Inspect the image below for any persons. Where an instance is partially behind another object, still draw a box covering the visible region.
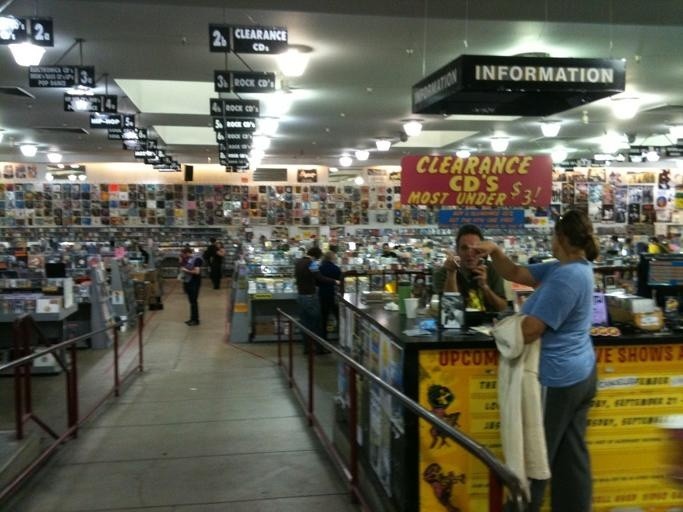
[317,251,345,338]
[215,241,225,279]
[176,246,203,326]
[293,246,340,356]
[381,242,397,257]
[203,237,224,289]
[465,207,602,512]
[432,224,508,314]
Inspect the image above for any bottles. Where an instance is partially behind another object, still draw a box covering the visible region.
[397,280,411,314]
[430,294,439,311]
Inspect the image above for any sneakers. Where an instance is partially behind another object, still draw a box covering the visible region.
[185,319,200,326]
[316,348,331,355]
[305,349,313,355]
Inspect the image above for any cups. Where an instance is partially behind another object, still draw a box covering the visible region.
[402,297,420,319]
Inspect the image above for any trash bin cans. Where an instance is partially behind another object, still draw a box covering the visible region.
[63,303,91,348]
